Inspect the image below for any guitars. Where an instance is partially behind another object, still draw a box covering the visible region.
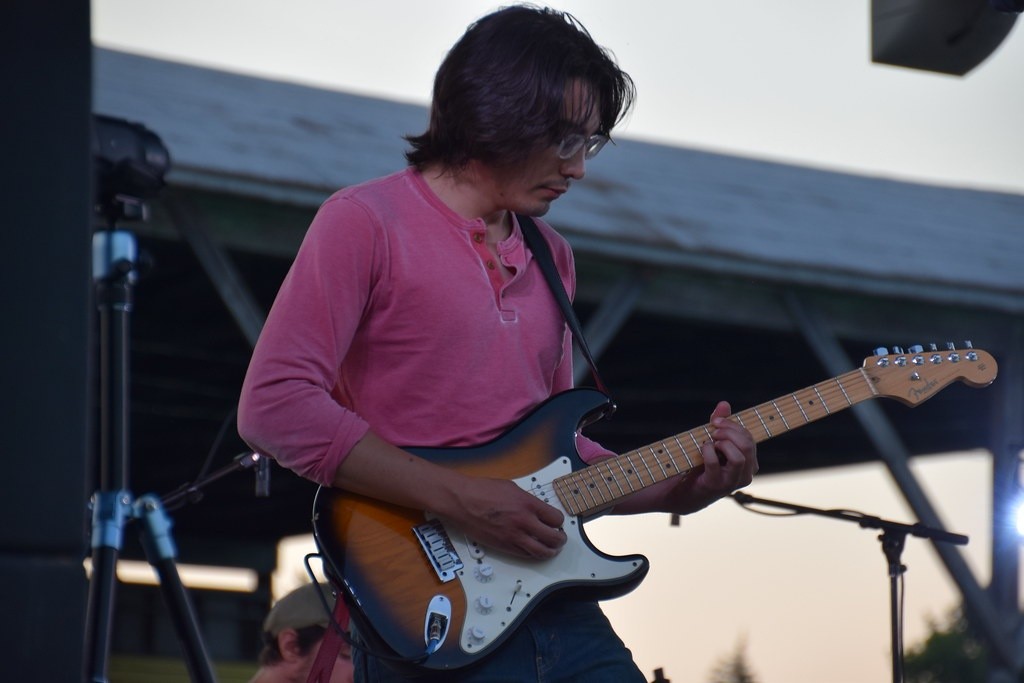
[312,340,997,681]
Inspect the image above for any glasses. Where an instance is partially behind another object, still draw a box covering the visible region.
[556,133,608,159]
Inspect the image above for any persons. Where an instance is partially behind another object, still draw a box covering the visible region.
[219,4,763,683]
[239,578,375,682]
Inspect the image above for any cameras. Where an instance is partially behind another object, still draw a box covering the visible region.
[89,113,168,209]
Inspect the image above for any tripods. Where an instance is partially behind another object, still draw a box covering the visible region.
[81,230,220,683]
